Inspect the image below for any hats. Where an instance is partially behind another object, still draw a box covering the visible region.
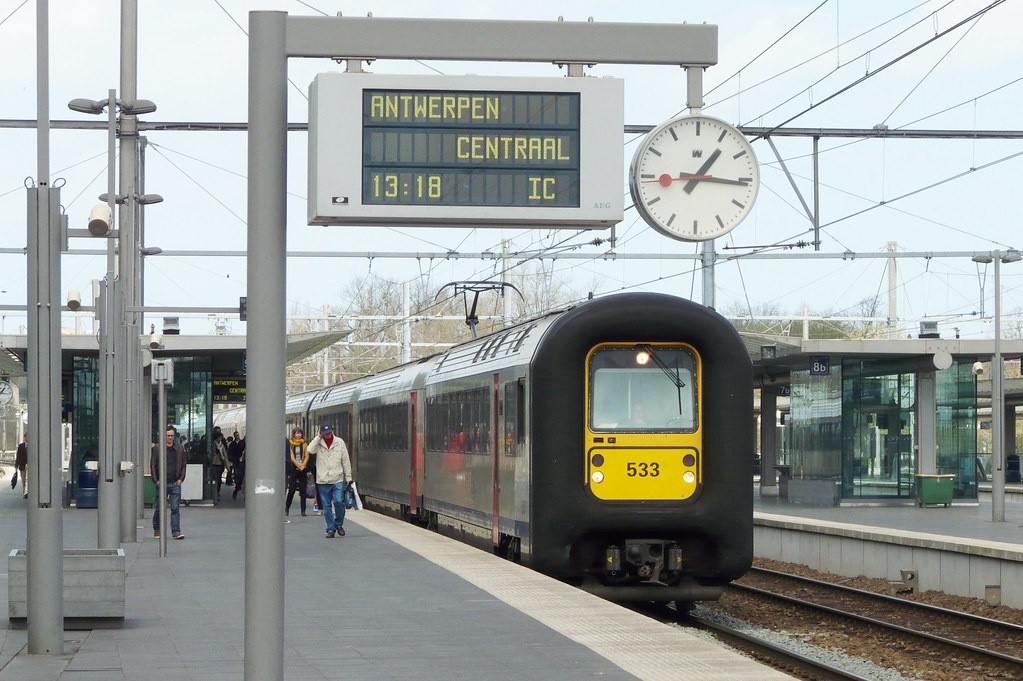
[320,423,332,434]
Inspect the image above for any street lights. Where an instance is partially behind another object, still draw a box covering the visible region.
[67,88,165,552]
[972,248,1022,522]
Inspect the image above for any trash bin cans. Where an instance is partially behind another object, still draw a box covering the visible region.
[143,476,156,508]
[914,473,956,508]
[753,458,761,474]
[1007,455,1021,482]
[76,470,98,509]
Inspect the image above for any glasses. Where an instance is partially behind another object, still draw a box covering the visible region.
[167,435,173,437]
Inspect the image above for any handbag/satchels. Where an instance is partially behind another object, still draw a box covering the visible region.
[226,471,233,486]
[350,481,363,511]
[239,452,245,475]
[345,482,354,509]
[11,472,17,489]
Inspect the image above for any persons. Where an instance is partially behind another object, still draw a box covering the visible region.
[15,432,28,498]
[308,453,324,516]
[151,427,186,539]
[214,426,245,501]
[306,425,353,538]
[175,429,209,483]
[285,427,311,516]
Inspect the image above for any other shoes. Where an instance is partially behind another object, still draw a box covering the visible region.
[233,492,236,499]
[23,495,27,498]
[285,511,289,516]
[313,504,318,511]
[301,511,306,516]
[316,510,324,515]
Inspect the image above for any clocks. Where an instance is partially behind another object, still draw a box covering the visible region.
[630,113,761,242]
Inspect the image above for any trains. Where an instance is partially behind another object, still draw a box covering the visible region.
[176,281,755,618]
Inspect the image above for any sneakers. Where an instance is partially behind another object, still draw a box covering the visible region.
[173,531,184,539]
[154,530,160,538]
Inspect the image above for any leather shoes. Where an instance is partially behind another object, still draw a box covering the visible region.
[336,527,345,536]
[325,532,334,538]
